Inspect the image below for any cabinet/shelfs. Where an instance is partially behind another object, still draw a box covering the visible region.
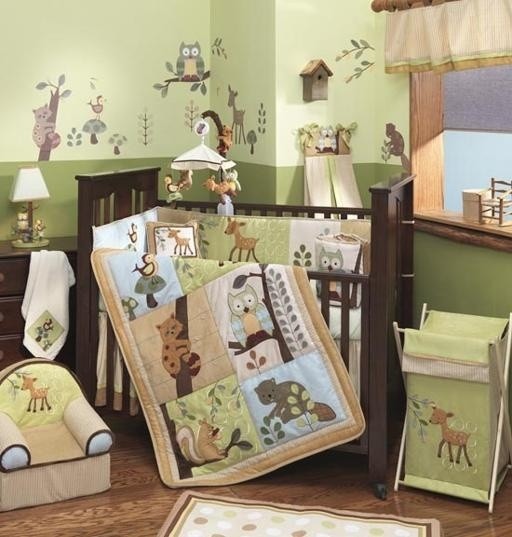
[1,234,77,374]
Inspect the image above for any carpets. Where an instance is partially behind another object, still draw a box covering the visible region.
[158,491,440,536]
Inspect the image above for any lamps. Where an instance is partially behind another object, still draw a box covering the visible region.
[9,162,52,249]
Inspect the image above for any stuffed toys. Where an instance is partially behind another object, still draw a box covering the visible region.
[163,119,242,205]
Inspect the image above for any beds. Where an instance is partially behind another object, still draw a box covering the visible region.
[74,166,415,503]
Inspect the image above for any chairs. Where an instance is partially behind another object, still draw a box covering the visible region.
[476,178,512,228]
[0,360,113,512]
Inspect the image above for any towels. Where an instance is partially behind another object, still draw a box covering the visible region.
[20,249,76,363]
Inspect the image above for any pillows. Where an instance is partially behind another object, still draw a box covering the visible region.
[145,217,203,262]
